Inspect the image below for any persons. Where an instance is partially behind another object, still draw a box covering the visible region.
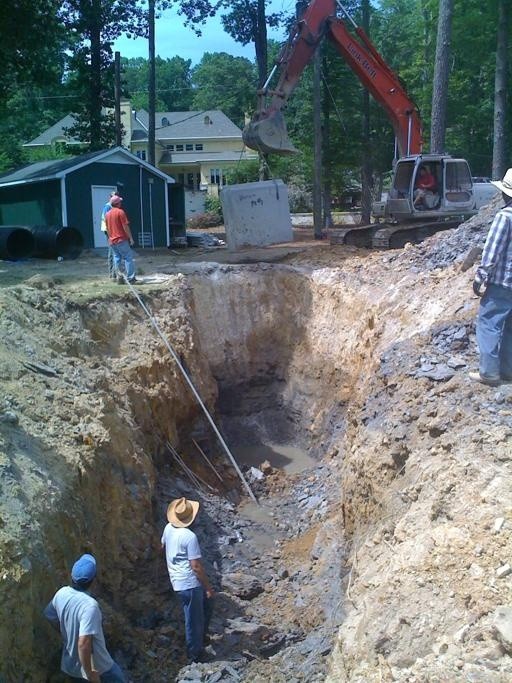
[42,554,128,683]
[413,166,435,207]
[101,191,119,282]
[160,495,216,667]
[105,194,137,285]
[467,168,512,388]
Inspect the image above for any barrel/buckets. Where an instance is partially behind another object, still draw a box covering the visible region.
[188,236,201,247]
[30,225,85,259]
[0,227,33,261]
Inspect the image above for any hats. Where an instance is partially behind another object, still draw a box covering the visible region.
[166,496,199,527]
[109,195,122,204]
[70,553,97,583]
[489,167,512,197]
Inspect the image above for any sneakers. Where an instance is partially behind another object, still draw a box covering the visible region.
[117,278,124,284]
[187,652,215,664]
[469,371,501,383]
[129,277,142,284]
[203,634,210,646]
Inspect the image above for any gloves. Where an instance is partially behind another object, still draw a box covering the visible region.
[473,281,482,296]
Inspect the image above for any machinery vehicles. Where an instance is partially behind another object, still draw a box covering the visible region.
[242,1,480,250]
